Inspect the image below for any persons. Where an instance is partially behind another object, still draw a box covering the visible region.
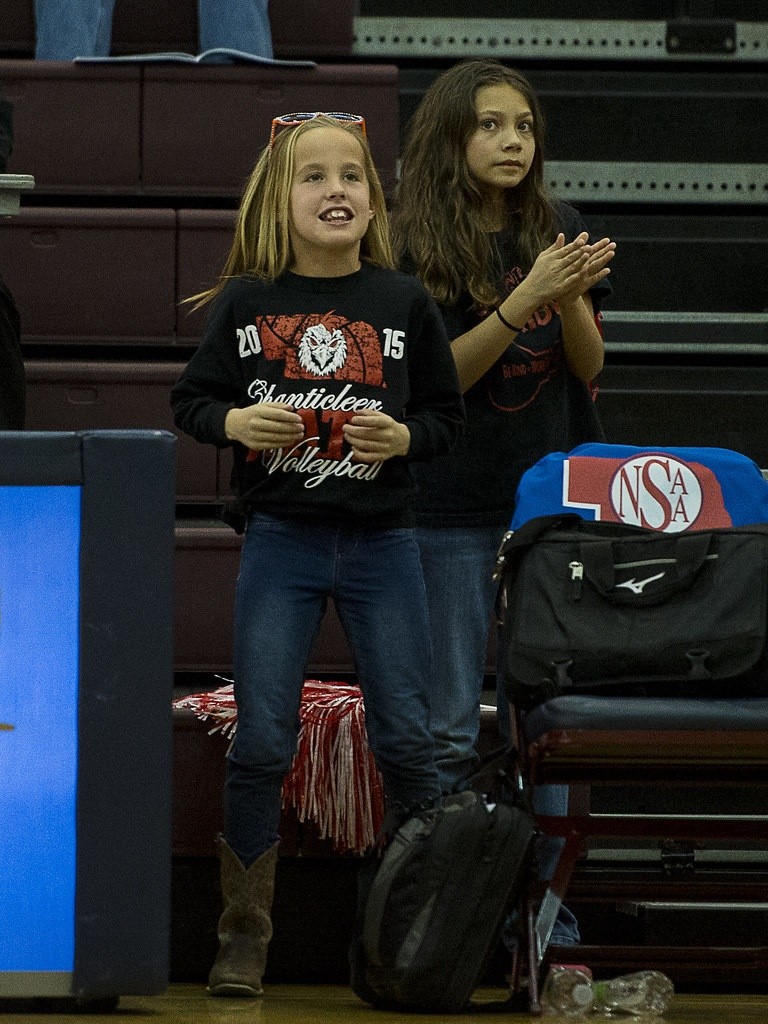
[162,110,466,997]
[33,0,275,65]
[386,63,617,1012]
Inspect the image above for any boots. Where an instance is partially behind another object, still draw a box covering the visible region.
[204,834,281,996]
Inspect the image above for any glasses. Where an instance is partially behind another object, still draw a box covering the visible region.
[268,112,367,159]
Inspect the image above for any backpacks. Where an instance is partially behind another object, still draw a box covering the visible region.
[349,790,536,1016]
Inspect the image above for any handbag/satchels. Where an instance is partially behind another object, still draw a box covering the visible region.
[490,513,768,744]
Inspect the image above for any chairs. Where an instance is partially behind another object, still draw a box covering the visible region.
[500,443,768,1016]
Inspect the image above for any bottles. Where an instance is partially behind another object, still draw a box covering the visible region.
[593,970,673,1017]
[539,967,595,1018]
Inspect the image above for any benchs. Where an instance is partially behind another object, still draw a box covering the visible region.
[0,0,768,857]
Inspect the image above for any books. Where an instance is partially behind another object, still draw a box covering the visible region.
[69,47,318,69]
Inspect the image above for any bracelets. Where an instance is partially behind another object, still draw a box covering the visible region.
[495,307,524,332]
